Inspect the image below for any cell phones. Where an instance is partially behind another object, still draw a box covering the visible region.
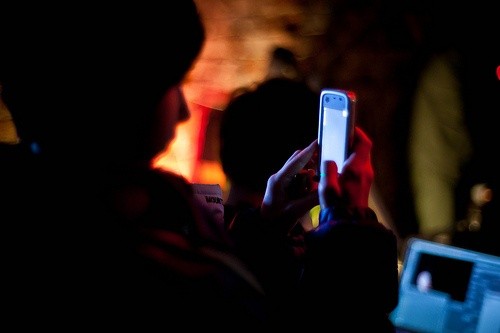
[316,89,357,180]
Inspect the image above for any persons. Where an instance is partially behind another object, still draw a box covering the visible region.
[0,0,400,333]
[219,78,319,234]
